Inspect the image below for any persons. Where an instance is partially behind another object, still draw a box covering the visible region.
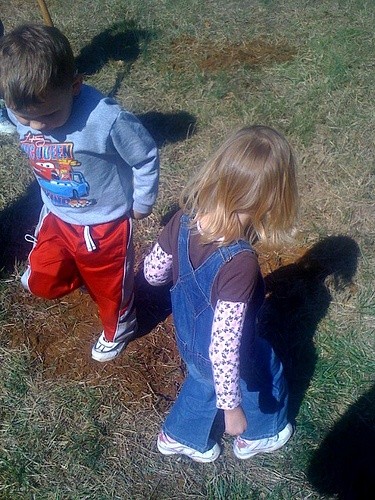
[0,21,161,363]
[143,124,300,464]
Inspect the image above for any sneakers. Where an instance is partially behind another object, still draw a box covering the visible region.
[232,424,295,459]
[157,428,221,462]
[91,330,128,361]
